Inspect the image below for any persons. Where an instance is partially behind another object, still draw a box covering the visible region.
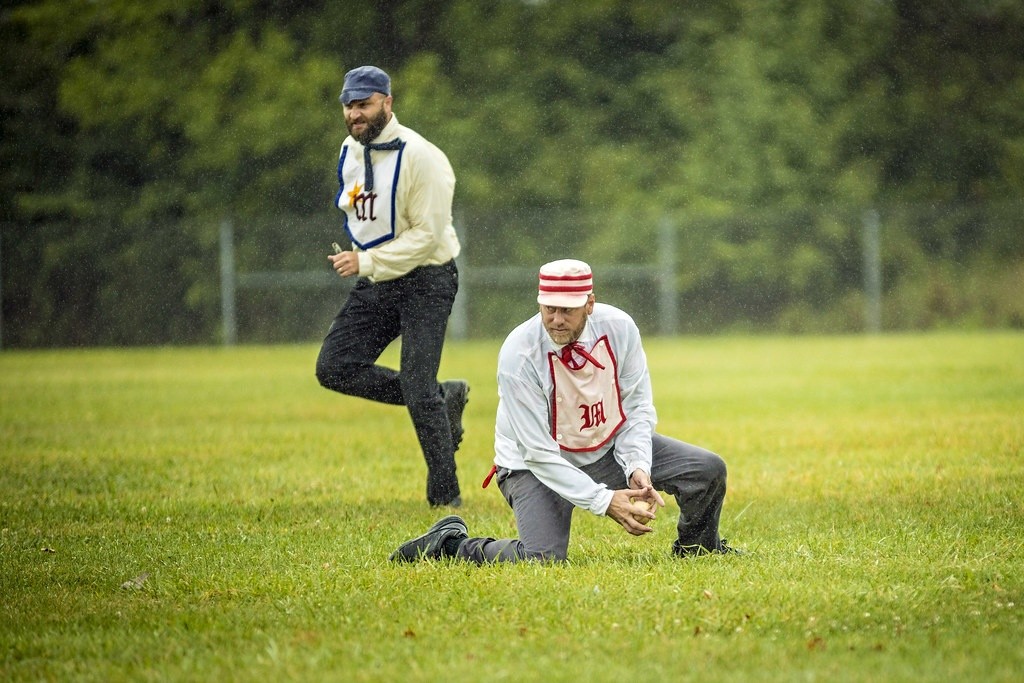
[387,259,743,563]
[316,64,470,514]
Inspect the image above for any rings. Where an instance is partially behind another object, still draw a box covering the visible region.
[339,268,343,273]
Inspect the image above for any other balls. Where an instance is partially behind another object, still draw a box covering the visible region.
[633,500,652,523]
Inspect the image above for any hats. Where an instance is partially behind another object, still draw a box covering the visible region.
[338,66,391,104]
[537,259,593,308]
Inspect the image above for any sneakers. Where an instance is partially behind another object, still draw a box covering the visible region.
[671,539,746,559]
[389,515,469,566]
[440,380,470,453]
